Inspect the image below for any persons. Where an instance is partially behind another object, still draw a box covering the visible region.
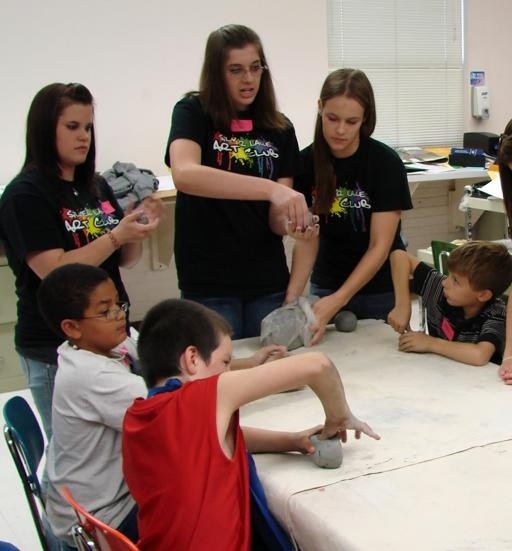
[498,118,512,385]
[36,263,293,551]
[388,241,512,366]
[0,82,165,448]
[283,68,413,350]
[121,298,381,551]
[163,22,320,340]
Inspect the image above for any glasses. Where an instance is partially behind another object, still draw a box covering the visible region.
[223,63,271,80]
[72,300,130,322]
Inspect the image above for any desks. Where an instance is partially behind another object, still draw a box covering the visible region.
[214,314,512,550]
[416,238,470,330]
[396,146,499,198]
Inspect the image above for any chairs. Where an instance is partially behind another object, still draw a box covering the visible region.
[2,395,50,551]
[61,485,139,550]
[430,240,458,276]
